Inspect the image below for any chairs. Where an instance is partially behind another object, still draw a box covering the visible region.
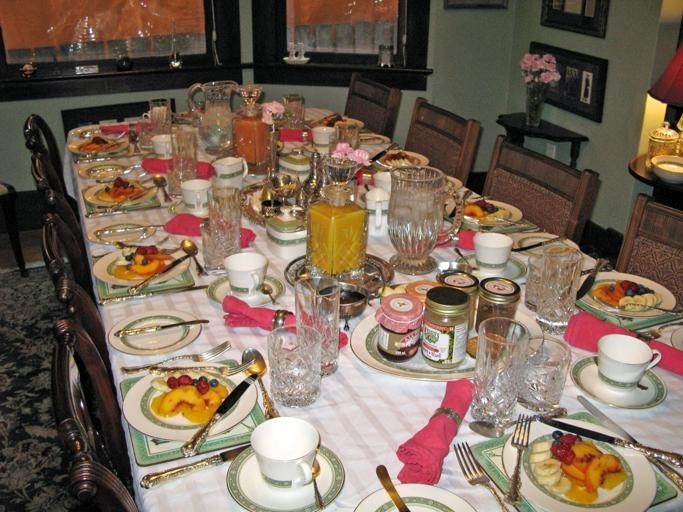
[615,192,683,308]
[405,97,482,184]
[61,100,176,144]
[0,182,31,278]
[482,133,601,249]
[344,70,402,145]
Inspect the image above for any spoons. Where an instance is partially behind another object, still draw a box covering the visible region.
[241,349,278,421]
[310,459,325,512]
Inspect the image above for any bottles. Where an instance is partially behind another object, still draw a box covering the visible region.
[377,270,521,369]
[309,155,369,274]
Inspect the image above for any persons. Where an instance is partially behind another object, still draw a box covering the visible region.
[582,73,591,102]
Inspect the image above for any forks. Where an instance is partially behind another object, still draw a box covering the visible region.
[509,414,532,506]
[454,442,510,511]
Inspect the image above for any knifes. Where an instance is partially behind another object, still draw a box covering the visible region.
[114,319,214,338]
[576,392,681,462]
[536,411,681,463]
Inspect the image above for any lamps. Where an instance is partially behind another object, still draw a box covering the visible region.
[650,37,683,159]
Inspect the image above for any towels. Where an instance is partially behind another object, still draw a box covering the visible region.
[275,125,313,142]
[395,379,476,486]
[222,295,349,350]
[162,212,255,252]
[403,229,476,251]
[354,166,377,189]
[143,155,215,180]
[102,120,160,139]
[564,307,682,378]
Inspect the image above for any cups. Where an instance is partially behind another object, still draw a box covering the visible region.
[143,79,307,270]
[328,123,362,153]
[468,317,573,426]
[385,167,445,274]
[518,234,584,335]
[266,275,342,408]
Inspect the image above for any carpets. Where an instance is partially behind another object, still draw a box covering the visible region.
[0,251,134,512]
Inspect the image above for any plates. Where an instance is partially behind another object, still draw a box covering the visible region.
[570,269,682,334]
[108,307,202,354]
[304,117,431,173]
[81,182,162,217]
[573,354,668,410]
[286,253,394,293]
[470,411,677,512]
[510,233,580,257]
[349,310,544,383]
[67,131,152,182]
[353,483,479,512]
[119,359,269,468]
[208,274,285,307]
[446,199,540,234]
[87,217,157,244]
[456,252,528,281]
[91,249,195,300]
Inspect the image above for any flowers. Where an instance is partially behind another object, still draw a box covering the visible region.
[519,54,561,126]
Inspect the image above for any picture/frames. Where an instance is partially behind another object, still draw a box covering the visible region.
[531,0,611,119]
[442,1,509,10]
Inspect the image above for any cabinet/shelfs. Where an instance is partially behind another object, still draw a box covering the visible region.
[497,111,590,167]
[629,149,682,208]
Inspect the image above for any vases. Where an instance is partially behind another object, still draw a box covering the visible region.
[526,84,548,126]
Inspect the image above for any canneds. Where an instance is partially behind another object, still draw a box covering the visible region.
[377,293,423,363]
[475,276,521,334]
[405,280,445,347]
[421,286,470,369]
[435,270,479,332]
